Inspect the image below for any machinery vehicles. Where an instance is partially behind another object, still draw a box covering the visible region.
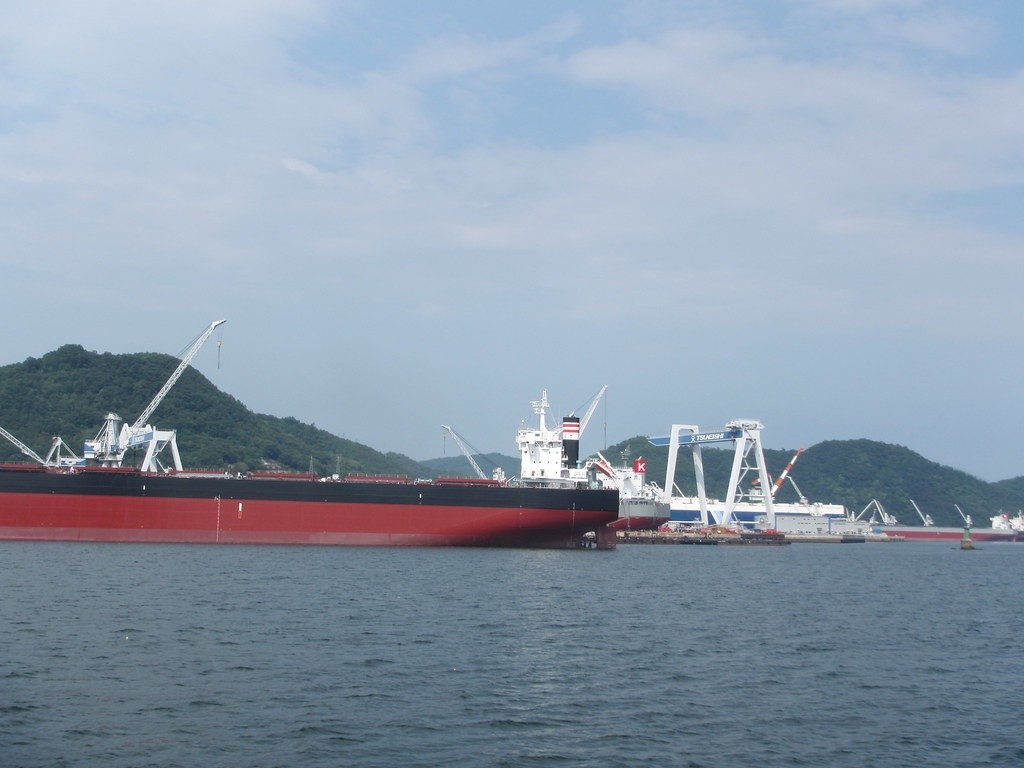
[1,318,228,468]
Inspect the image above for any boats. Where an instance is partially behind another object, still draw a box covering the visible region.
[1,461,618,546]
